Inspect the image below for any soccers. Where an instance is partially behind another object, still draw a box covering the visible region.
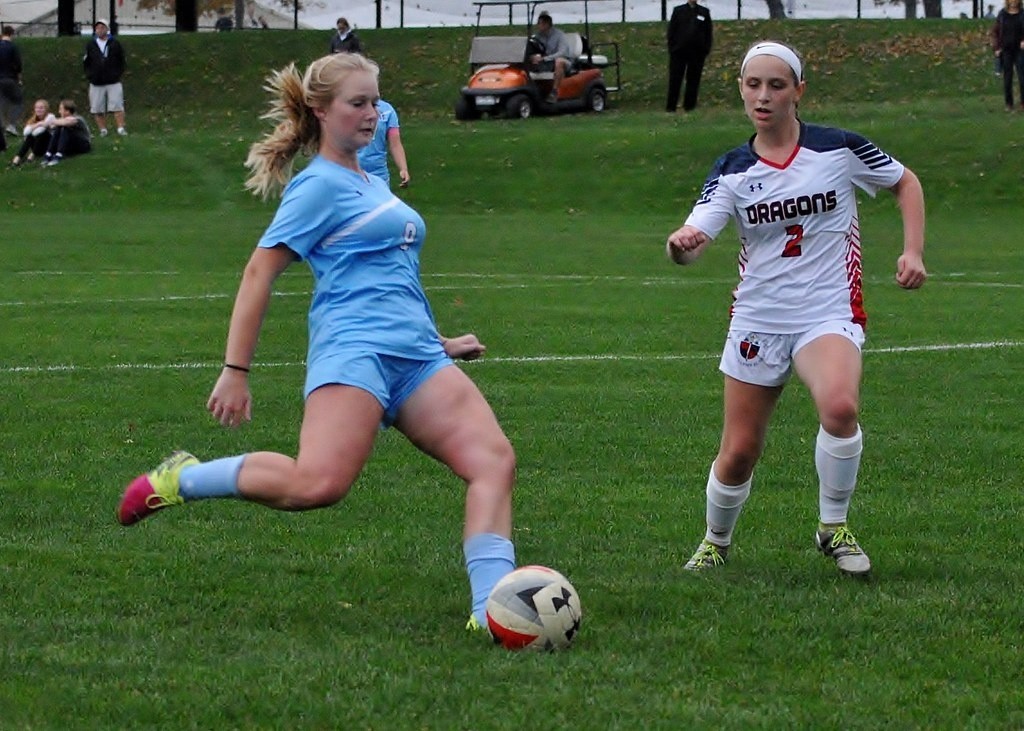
[486,564,585,651]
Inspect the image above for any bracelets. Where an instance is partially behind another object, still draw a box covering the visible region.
[223,363,249,372]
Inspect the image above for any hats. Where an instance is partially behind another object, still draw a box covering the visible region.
[95,19,111,31]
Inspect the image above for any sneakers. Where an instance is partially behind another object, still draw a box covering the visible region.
[686,541,729,572]
[815,519,871,576]
[116,450,200,525]
[467,613,492,638]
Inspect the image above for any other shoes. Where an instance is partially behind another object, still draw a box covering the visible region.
[547,92,557,104]
[100,131,109,137]
[5,162,20,171]
[1004,105,1013,113]
[119,130,128,136]
[42,158,61,167]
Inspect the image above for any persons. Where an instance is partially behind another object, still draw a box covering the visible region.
[0,25,25,136]
[986,4,996,17]
[666,0,713,113]
[530,13,575,104]
[8,100,56,167]
[663,42,927,572]
[993,0,1024,111]
[356,99,410,190]
[115,53,518,636]
[82,18,128,137]
[330,18,361,53]
[39,99,92,167]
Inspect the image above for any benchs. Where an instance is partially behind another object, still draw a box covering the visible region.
[530,33,582,79]
[577,31,609,66]
